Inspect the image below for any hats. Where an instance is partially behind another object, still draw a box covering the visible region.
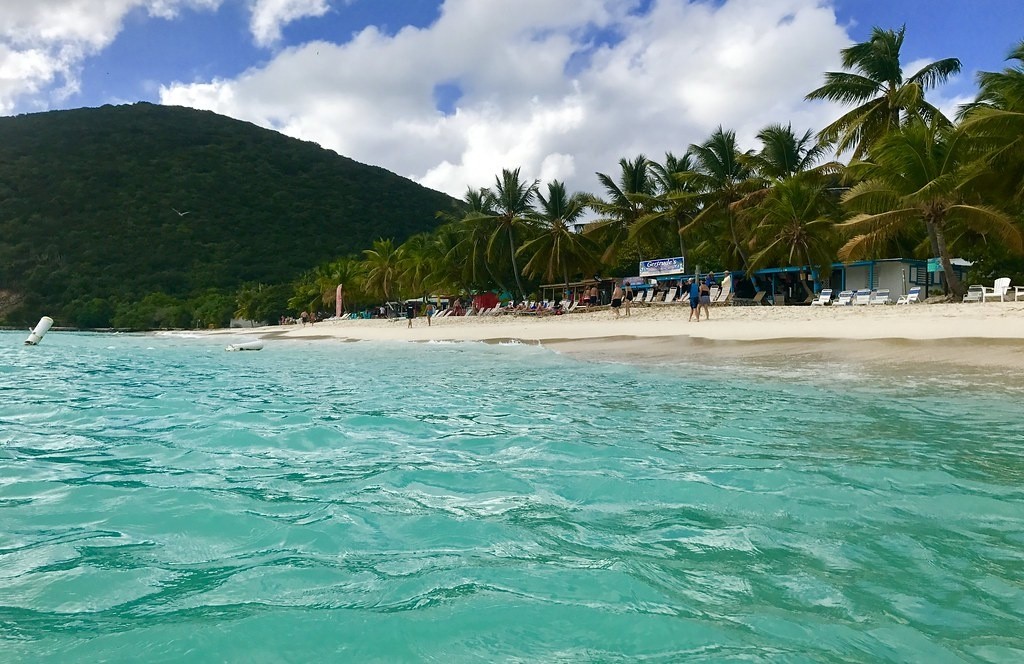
[723,270,731,274]
[689,278,694,281]
[708,271,715,275]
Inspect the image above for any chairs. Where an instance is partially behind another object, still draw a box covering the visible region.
[853,288,872,306]
[809,289,832,306]
[321,298,580,325]
[1012,285,1024,302]
[832,290,855,307]
[983,277,1012,303]
[895,286,922,306]
[736,290,766,306]
[871,289,891,305]
[962,284,984,304]
[601,283,736,308]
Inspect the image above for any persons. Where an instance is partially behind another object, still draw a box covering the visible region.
[721,270,730,288]
[423,301,433,326]
[706,271,716,287]
[582,286,598,313]
[380,306,386,318]
[406,301,415,329]
[502,301,563,312]
[610,282,623,319]
[300,310,316,327]
[624,282,633,316]
[454,297,462,316]
[681,278,710,322]
